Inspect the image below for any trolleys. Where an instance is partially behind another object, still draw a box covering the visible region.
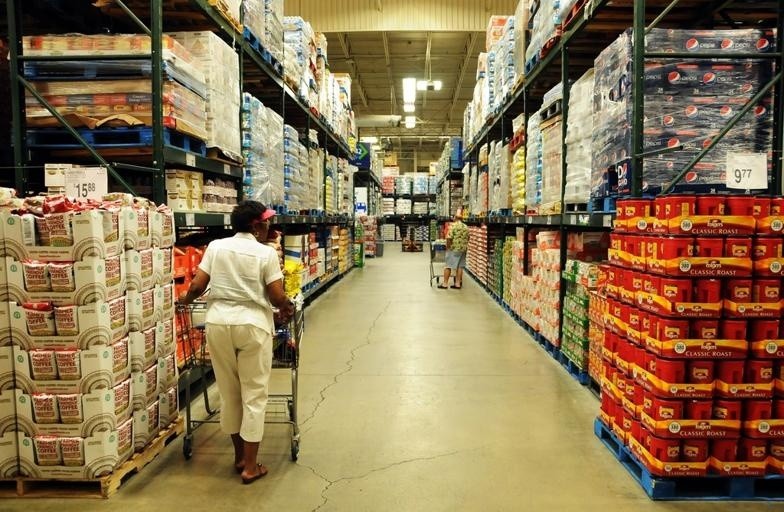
[428,238,457,287]
[173,290,305,465]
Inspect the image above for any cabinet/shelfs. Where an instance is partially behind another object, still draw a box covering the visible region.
[381,174,437,243]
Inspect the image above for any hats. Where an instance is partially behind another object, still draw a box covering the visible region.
[245,208,276,226]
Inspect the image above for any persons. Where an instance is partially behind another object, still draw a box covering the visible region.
[437,213,470,289]
[177,200,297,484]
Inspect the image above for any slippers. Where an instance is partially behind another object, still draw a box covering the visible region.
[450,283,461,289]
[235,458,245,474]
[242,464,269,484]
[437,283,448,289]
